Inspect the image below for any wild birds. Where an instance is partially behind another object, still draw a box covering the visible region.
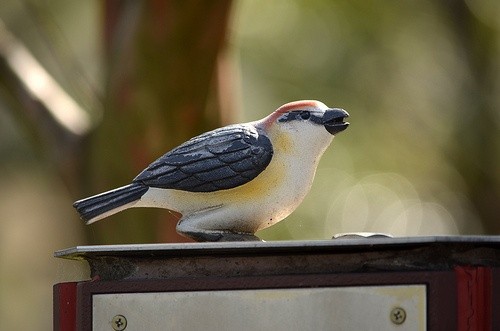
[73,99,351,242]
